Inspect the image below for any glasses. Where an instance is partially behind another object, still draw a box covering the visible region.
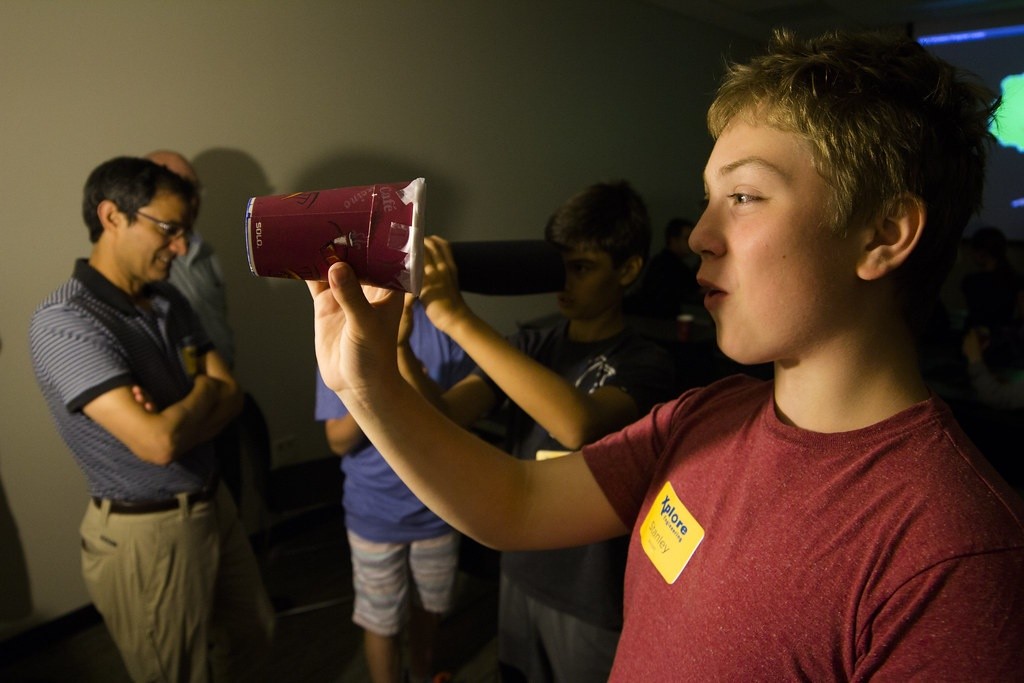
[134,209,194,244]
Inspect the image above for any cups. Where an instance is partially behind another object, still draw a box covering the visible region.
[677,315,694,340]
[246,178,426,296]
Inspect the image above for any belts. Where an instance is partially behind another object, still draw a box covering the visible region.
[92,472,220,514]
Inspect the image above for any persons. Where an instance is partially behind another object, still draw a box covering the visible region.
[29,148,277,683]
[302,24,1024,683]
